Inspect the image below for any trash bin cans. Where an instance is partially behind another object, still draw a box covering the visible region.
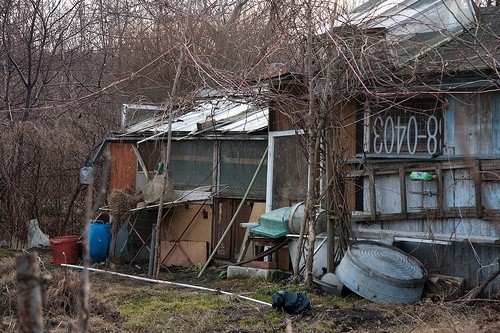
[82,220,113,263]
[49,235,79,265]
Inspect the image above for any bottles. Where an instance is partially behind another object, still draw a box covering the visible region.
[410,172,437,181]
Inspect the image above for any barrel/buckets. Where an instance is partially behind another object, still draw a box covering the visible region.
[82,219,110,263]
[282,201,328,236]
[49,235,78,265]
